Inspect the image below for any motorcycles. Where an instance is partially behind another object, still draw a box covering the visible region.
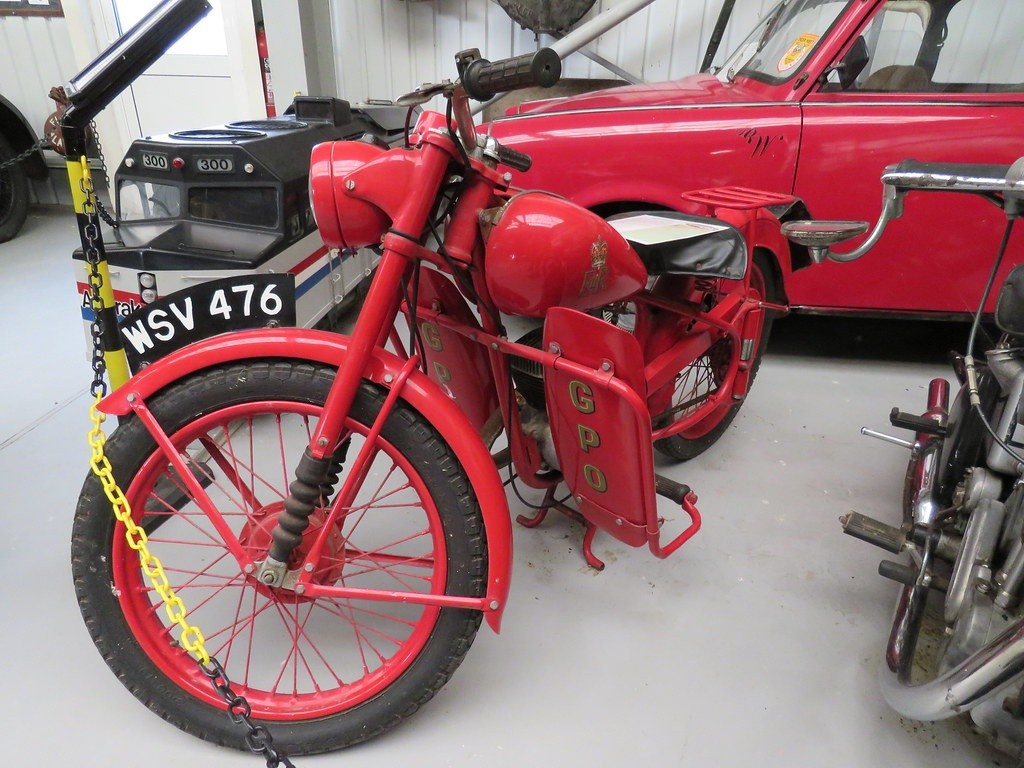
[837,156,1024,729]
[65,46,819,757]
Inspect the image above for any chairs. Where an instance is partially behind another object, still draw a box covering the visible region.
[859,64,928,92]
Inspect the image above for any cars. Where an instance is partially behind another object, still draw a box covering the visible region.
[440,0,1024,328]
